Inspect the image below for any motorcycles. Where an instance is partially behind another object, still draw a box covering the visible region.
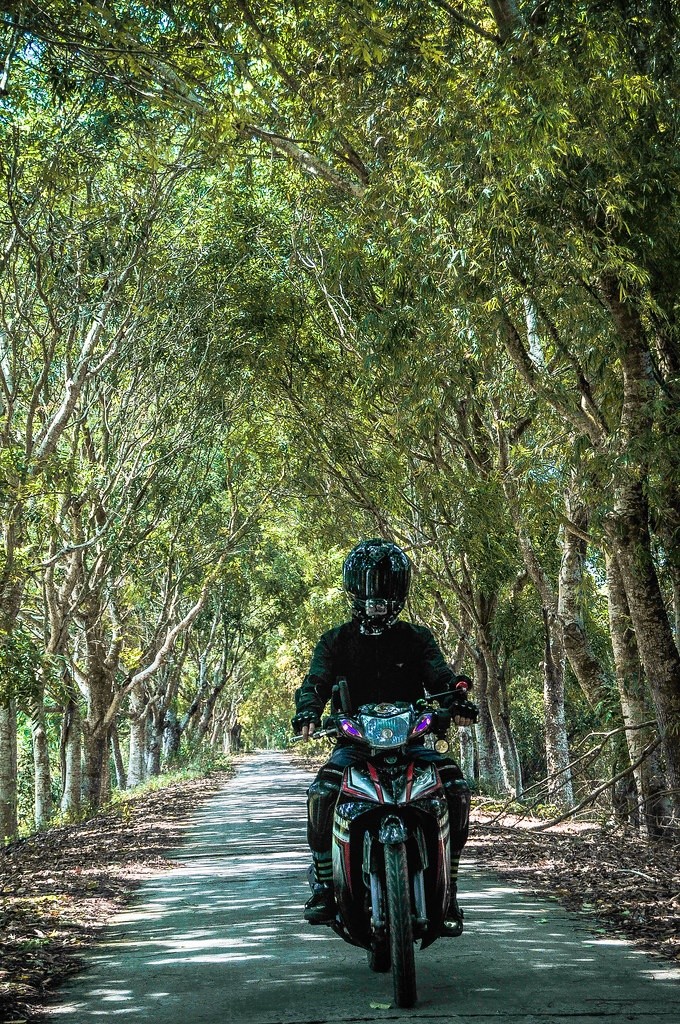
[290,685,481,1009]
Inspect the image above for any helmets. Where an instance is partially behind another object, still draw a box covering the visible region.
[342,537,412,634]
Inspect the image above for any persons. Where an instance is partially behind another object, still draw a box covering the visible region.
[292,538,478,938]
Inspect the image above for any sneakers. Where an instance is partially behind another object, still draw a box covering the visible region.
[445,909,464,937]
[303,895,339,921]
